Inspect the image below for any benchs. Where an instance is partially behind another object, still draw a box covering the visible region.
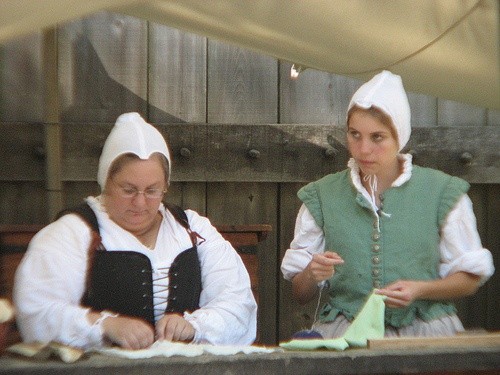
[0,221,272,355]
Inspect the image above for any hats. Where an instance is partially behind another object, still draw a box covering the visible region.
[95,111,172,195]
[345,69,413,153]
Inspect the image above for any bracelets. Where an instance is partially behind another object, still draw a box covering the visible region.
[93,310,121,329]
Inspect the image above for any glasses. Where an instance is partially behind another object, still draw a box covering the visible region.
[109,176,166,199]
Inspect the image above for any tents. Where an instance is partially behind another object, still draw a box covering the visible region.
[1,0,500,224]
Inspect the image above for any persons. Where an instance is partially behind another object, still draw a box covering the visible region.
[280,70,496,342]
[15,110,260,356]
